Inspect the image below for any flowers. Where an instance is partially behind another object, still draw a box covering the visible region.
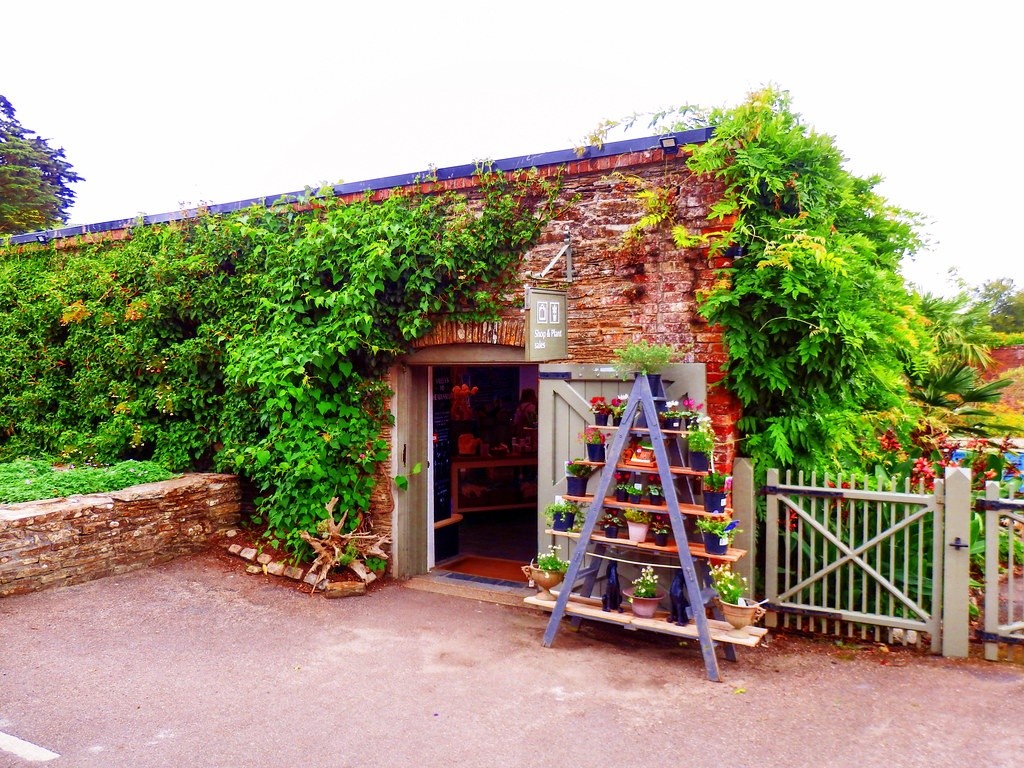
[537,395,750,604]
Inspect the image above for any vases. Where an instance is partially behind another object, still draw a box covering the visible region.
[518,411,767,639]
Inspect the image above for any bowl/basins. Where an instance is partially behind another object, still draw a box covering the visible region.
[488,450,509,457]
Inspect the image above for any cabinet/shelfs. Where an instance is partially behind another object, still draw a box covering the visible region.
[450,452,537,513]
[524,373,769,682]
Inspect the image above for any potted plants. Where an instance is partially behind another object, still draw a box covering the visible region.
[610,339,693,398]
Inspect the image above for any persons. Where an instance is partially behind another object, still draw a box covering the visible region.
[513,389,537,436]
